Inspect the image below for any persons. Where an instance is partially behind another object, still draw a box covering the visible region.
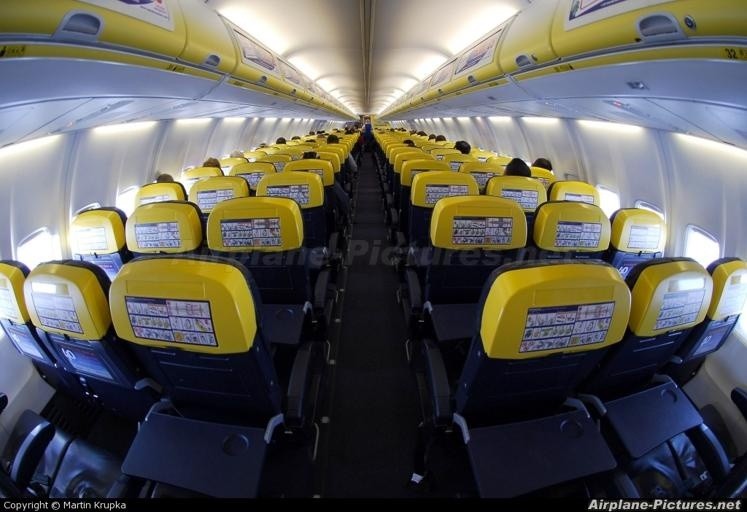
[156,120,365,207]
[374,125,553,178]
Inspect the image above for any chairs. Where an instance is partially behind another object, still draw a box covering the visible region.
[0,126,747,512]
